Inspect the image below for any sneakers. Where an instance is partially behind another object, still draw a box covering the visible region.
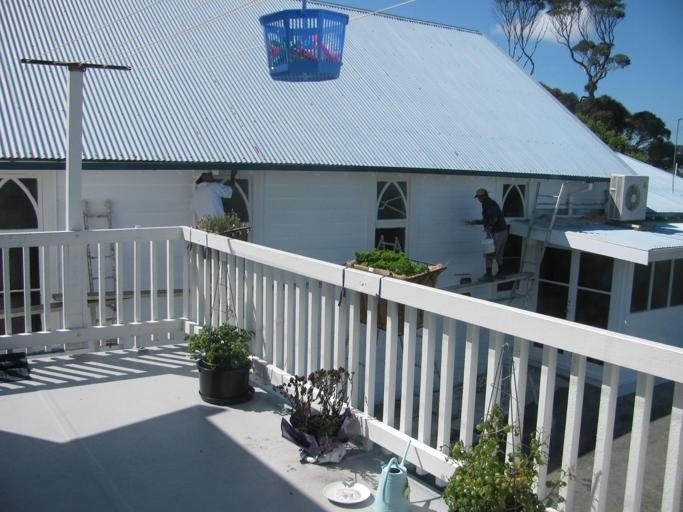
[495,271,506,279]
[478,273,494,282]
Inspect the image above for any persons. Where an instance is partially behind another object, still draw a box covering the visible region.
[461,187,510,282]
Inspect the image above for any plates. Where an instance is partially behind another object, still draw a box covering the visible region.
[322,481,371,504]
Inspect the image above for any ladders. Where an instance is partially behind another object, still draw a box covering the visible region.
[507,181,566,310]
[83,199,118,325]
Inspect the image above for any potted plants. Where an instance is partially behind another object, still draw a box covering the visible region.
[268,365,357,465]
[184,210,257,404]
[341,249,448,336]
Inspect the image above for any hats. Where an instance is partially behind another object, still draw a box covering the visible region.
[473,188,487,199]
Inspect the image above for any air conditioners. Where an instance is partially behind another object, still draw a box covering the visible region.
[604,174,649,223]
